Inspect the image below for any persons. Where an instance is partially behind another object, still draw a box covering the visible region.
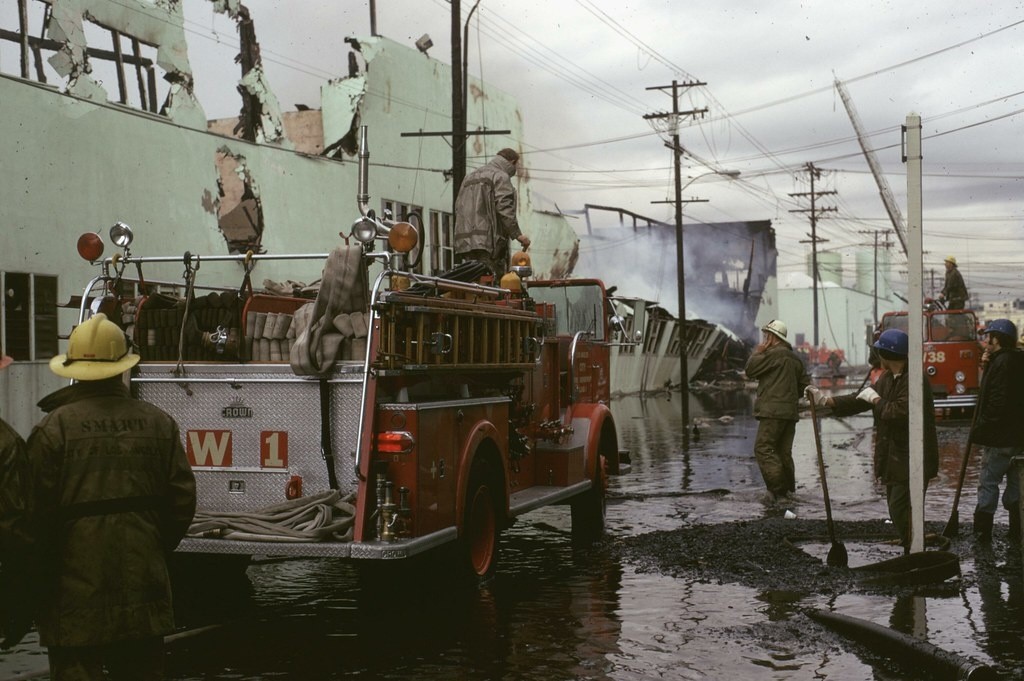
[971,319,1024,541]
[827,351,842,373]
[939,255,970,329]
[0,313,198,681]
[744,320,809,502]
[455,149,531,287]
[804,329,939,554]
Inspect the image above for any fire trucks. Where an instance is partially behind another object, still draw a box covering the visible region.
[49,123,649,588]
[865,306,984,421]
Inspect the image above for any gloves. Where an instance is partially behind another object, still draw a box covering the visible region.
[856,387,879,403]
[803,385,827,407]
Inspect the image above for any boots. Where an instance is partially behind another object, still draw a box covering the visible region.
[974,512,992,543]
[1008,508,1021,539]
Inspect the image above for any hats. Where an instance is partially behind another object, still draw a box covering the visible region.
[49,313,141,380]
[945,256,957,267]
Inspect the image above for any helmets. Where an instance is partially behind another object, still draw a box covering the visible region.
[762,320,790,345]
[874,329,908,354]
[983,319,1017,339]
